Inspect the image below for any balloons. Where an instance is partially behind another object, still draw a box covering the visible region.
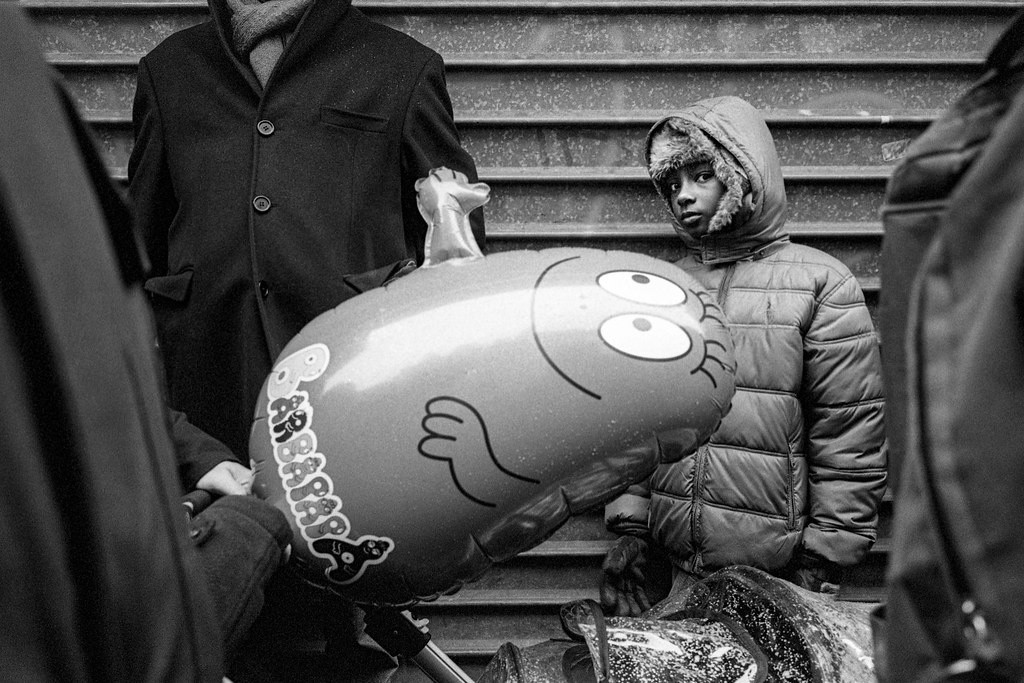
[249,163,736,611]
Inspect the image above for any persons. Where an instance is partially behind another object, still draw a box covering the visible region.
[121,0,492,464]
[598,96,885,615]
[869,12,1024,683]
[0,0,291,683]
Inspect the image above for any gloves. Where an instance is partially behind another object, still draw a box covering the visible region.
[599,531,655,618]
[792,549,842,592]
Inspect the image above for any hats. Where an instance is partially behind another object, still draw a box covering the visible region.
[649,118,755,235]
